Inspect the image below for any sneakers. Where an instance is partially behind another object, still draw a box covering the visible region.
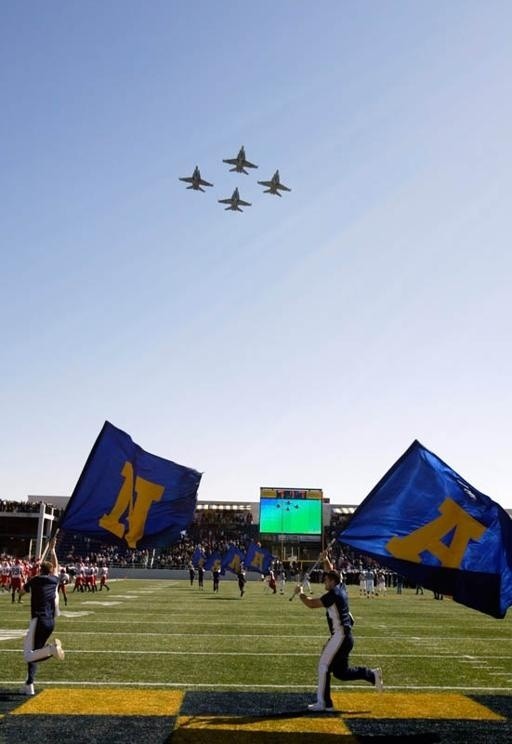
[18,684,36,696]
[190,582,314,598]
[360,592,445,600]
[0,586,111,604]
[307,702,334,712]
[371,667,385,691]
[51,638,65,660]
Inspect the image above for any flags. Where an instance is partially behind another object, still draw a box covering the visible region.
[335,437,512,619]
[54,419,204,551]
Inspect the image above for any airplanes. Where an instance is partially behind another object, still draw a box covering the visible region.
[180,143,292,215]
[275,499,299,514]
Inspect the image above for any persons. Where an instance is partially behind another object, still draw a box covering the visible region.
[67,508,254,597]
[1,499,68,604]
[330,549,444,600]
[260,568,313,595]
[294,549,385,713]
[17,535,67,695]
